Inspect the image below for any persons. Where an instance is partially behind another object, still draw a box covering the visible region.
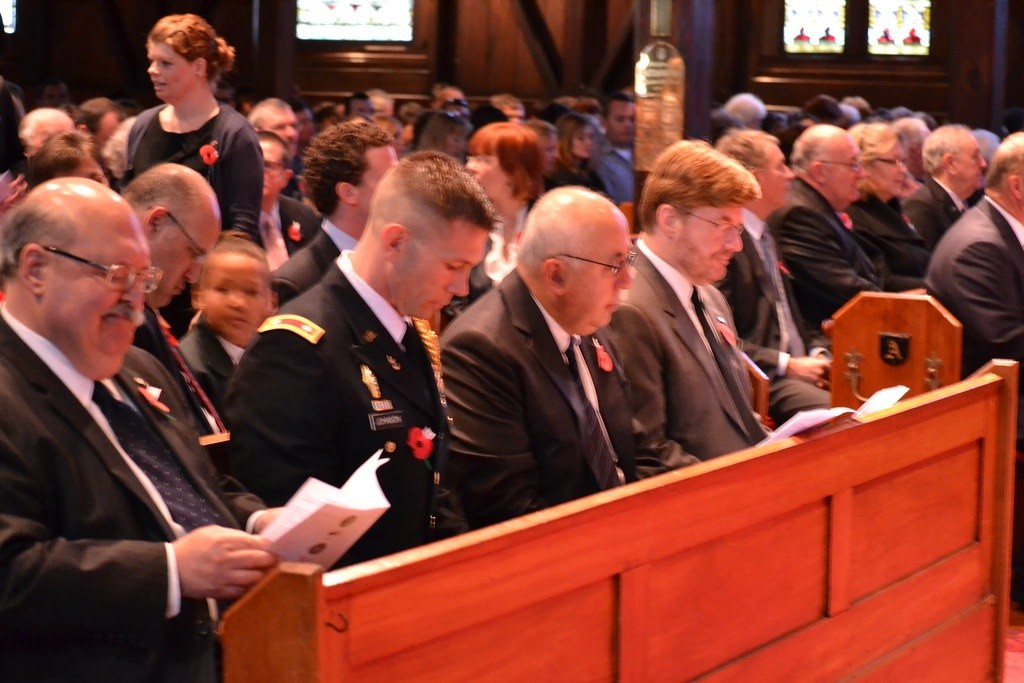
[0,77,504,682]
[118,12,265,341]
[779,93,1023,615]
[436,94,833,518]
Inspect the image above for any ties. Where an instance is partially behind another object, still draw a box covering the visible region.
[692,285,768,443]
[92,380,233,618]
[760,236,806,358]
[401,321,434,398]
[564,336,622,491]
[156,314,228,433]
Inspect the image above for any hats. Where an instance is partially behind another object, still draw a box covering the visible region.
[723,94,767,121]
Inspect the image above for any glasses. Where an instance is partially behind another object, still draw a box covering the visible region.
[813,156,860,172]
[667,202,745,238]
[542,251,638,277]
[14,244,164,292]
[147,207,206,264]
[875,157,909,169]
[263,161,288,171]
